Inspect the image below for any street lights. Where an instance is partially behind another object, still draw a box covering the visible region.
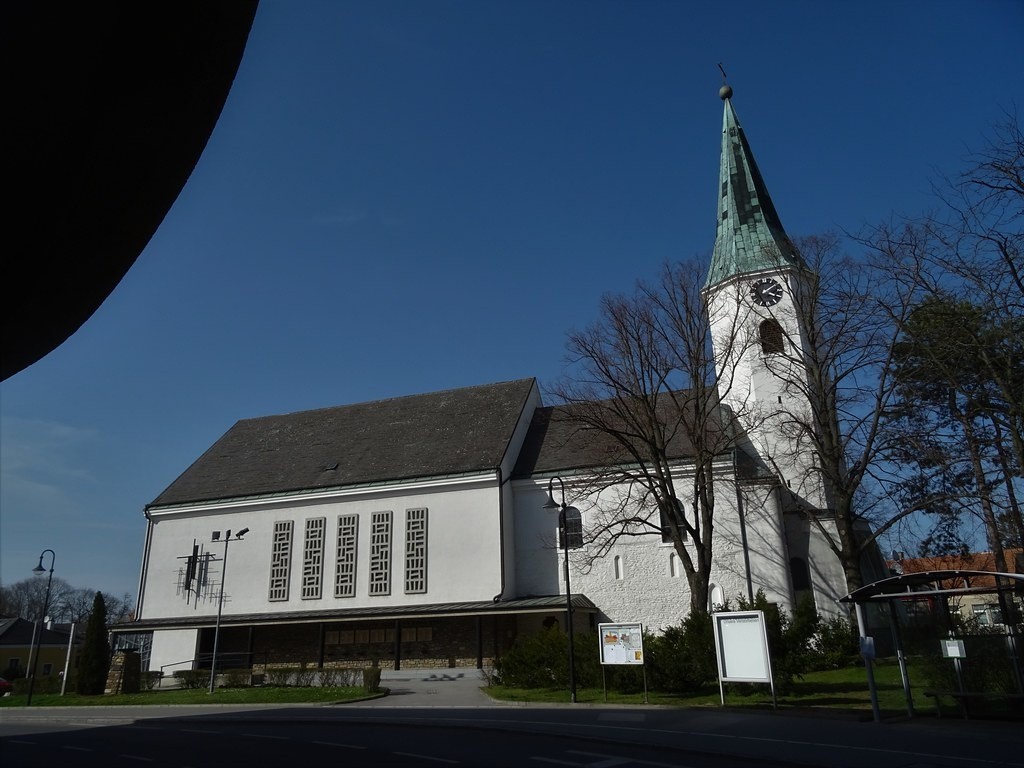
[210,528,250,694]
[26,549,55,706]
[542,475,576,703]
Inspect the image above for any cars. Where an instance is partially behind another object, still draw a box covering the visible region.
[0,677,15,697]
